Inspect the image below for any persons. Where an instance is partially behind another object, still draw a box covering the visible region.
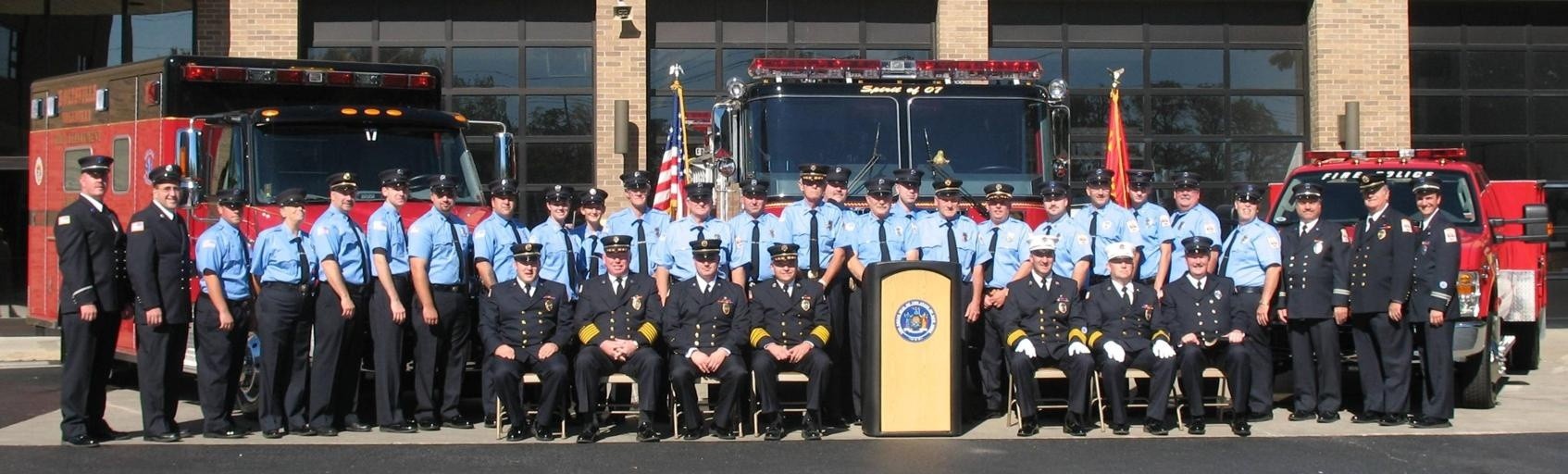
[651,182,750,305]
[746,244,832,440]
[1406,177,1460,429]
[124,163,192,441]
[1082,243,1177,436]
[1218,185,1283,421]
[480,243,574,441]
[50,154,133,447]
[1125,170,1175,300]
[773,164,929,424]
[308,172,371,435]
[966,183,1033,417]
[1001,236,1090,437]
[660,240,749,439]
[193,187,253,440]
[1163,171,1222,285]
[592,171,672,278]
[905,179,992,423]
[1275,182,1350,422]
[725,176,782,285]
[572,235,665,442]
[364,167,418,432]
[1075,167,1146,286]
[1160,237,1255,436]
[404,174,477,431]
[1030,181,1093,293]
[473,178,531,430]
[530,186,607,304]
[250,188,318,436]
[1350,173,1416,425]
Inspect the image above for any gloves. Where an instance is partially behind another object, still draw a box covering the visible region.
[1067,341,1091,357]
[1152,339,1177,360]
[1015,338,1037,359]
[1102,339,1126,364]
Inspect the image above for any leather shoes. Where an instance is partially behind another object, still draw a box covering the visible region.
[1064,424,1086,436]
[173,427,192,437]
[1380,415,1408,426]
[203,414,863,446]
[1351,415,1382,423]
[63,434,98,444]
[1017,426,1039,436]
[1113,424,1129,434]
[110,431,131,440]
[1289,414,1316,421]
[1144,421,1168,435]
[1409,416,1449,428]
[1232,417,1250,434]
[144,432,182,442]
[1189,419,1205,434]
[1317,415,1339,423]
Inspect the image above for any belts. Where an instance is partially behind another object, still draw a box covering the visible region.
[425,284,465,293]
[262,282,310,293]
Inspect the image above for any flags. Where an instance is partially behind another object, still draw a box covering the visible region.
[650,88,687,223]
[1104,88,1133,209]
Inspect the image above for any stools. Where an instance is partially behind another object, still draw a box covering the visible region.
[1094,367,1179,432]
[1007,367,1094,433]
[596,374,640,416]
[1177,368,1230,429]
[669,377,744,438]
[496,371,566,441]
[750,369,825,436]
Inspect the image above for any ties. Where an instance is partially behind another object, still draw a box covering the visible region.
[636,220,648,275]
[588,235,598,279]
[985,227,999,284]
[945,222,959,263]
[751,220,760,280]
[1368,218,1374,226]
[236,218,465,284]
[508,221,521,243]
[1197,281,1202,290]
[1041,278,1047,292]
[1300,224,1308,235]
[878,220,892,263]
[1121,287,1130,305]
[561,229,576,292]
[1133,210,1141,282]
[809,210,819,271]
[1045,225,1051,236]
[1090,211,1097,269]
[1218,230,1239,278]
[696,226,705,240]
[1170,215,1186,228]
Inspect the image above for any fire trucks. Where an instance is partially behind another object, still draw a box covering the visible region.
[674,50,1077,240]
[1261,146,1556,402]
[22,59,521,417]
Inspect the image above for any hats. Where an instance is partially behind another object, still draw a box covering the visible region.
[1230,183,1266,203]
[506,242,546,263]
[1171,173,1202,188]
[149,164,182,186]
[600,235,633,248]
[542,184,575,202]
[1038,181,1071,197]
[892,169,925,185]
[984,183,1014,201]
[378,168,411,187]
[216,190,249,205]
[276,188,307,206]
[799,165,830,183]
[425,174,461,191]
[79,156,114,173]
[683,182,715,198]
[1412,177,1442,195]
[1292,183,1325,200]
[689,240,724,253]
[864,178,895,195]
[739,179,770,194]
[326,172,361,191]
[579,188,608,205]
[619,170,652,190]
[827,167,851,184]
[1125,169,1155,189]
[768,245,801,256]
[932,181,963,196]
[1082,169,1114,184]
[488,179,519,198]
[1358,173,1387,194]
